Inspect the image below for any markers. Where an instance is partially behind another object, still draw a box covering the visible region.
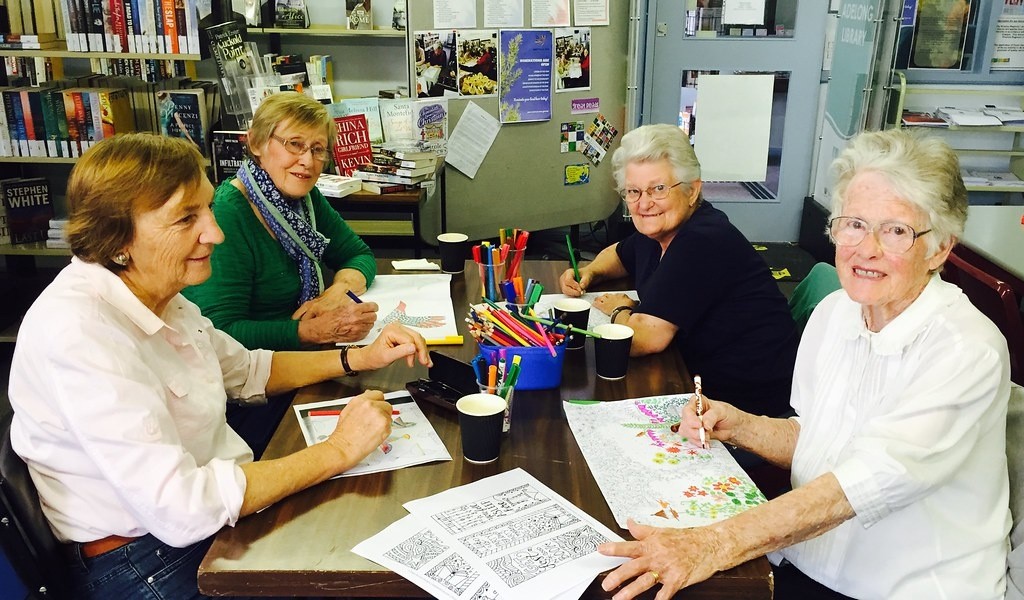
[346,288,365,302]
[504,282,518,313]
[524,283,542,316]
[307,410,404,415]
[498,280,508,302]
[473,228,528,303]
[512,277,524,305]
[473,349,522,432]
[694,374,706,448]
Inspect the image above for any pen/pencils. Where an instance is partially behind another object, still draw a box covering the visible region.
[565,237,584,296]
[522,278,540,314]
[466,295,602,357]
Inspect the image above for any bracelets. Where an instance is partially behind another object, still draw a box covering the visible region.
[611,306,634,325]
[340,344,360,378]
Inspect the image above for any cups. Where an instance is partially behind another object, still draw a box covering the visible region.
[477,244,535,313]
[552,298,591,350]
[436,232,469,274]
[476,373,519,432]
[455,393,508,464]
[593,323,635,381]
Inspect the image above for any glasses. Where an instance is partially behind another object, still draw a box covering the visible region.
[826,216,934,255]
[618,181,682,203]
[272,134,332,161]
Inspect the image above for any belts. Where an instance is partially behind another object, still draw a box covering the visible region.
[80,535,134,558]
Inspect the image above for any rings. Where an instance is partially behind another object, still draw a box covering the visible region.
[650,569,659,585]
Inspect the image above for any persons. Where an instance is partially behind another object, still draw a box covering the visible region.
[561,124,802,485]
[415,39,589,90]
[598,127,1013,600]
[178,91,378,444]
[9,133,432,600]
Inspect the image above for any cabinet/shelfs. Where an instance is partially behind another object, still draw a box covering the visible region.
[0,0,447,346]
[887,71,1024,200]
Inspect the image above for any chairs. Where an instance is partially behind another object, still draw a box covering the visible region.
[0,423,84,600]
[790,244,1024,600]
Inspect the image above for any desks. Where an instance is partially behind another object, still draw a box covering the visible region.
[196,258,773,600]
[958,205,1024,301]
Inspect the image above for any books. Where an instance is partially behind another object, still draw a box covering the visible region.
[961,169,1024,187]
[0,0,438,248]
